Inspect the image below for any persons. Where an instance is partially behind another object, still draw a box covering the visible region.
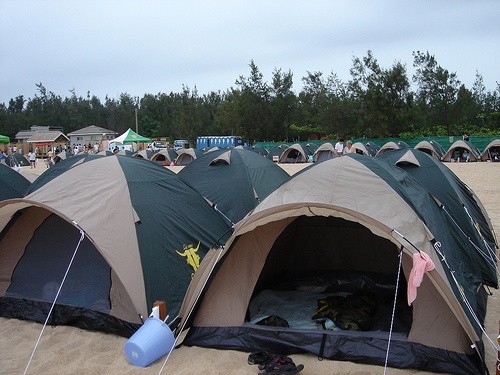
[224,144,251,151]
[458,150,470,163]
[343,140,356,155]
[47,142,101,160]
[234,138,249,147]
[0,149,7,164]
[28,147,40,169]
[335,138,344,155]
[8,145,22,156]
[111,146,125,155]
[307,154,314,163]
[462,133,469,142]
[490,149,499,161]
[148,141,156,148]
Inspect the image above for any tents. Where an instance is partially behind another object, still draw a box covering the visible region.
[375,147,500,290]
[0,135,11,146]
[0,154,235,349]
[247,139,500,163]
[96,150,114,157]
[177,146,291,223]
[173,153,491,375]
[6,153,30,166]
[115,150,133,158]
[131,146,223,167]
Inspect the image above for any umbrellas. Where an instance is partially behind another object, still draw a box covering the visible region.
[110,128,152,149]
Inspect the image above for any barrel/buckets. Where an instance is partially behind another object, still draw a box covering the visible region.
[124,317,175,367]
